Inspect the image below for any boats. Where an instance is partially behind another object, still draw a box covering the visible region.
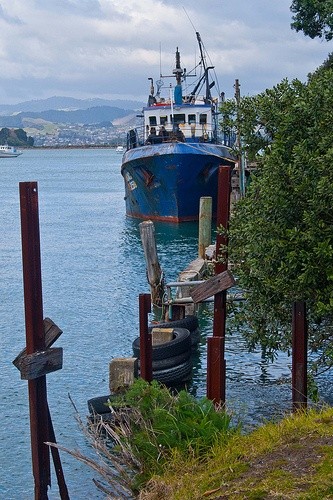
[115,147,123,154]
[122,32,242,225]
[1,146,24,159]
